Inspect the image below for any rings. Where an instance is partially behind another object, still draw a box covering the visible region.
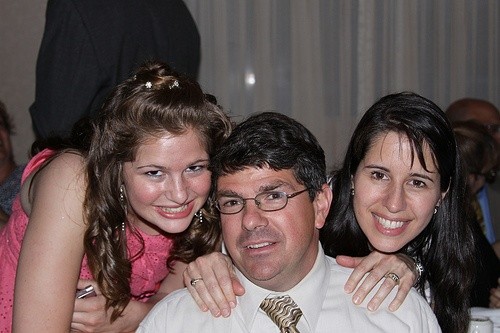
[190,277,203,286]
[385,272,400,285]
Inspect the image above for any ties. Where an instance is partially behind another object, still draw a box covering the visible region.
[258,294,302,333]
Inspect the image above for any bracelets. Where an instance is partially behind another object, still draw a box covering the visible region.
[396,252,424,287]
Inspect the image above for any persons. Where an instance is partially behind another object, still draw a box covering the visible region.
[0,0,500,333]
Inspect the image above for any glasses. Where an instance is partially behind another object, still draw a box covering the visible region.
[469,169,496,183]
[212,187,312,215]
